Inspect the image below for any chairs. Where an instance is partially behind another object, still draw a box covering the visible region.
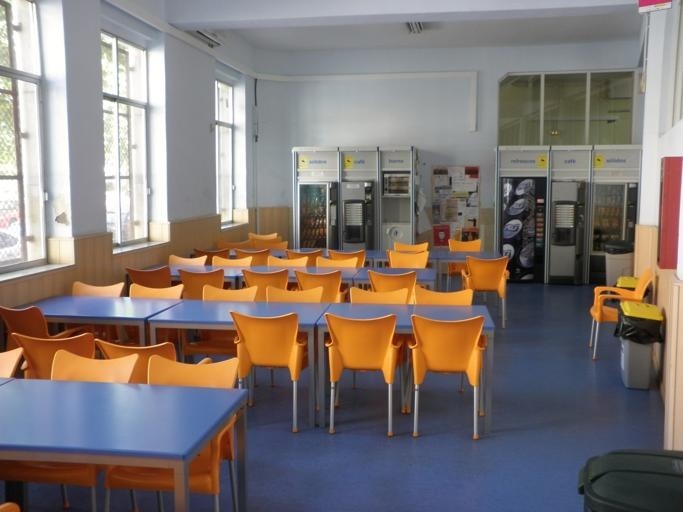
[589,268,655,360]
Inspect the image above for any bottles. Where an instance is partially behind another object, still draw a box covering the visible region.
[301,216,326,248]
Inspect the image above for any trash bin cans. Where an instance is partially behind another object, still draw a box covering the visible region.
[613,276,664,388]
[577,450,682,511]
